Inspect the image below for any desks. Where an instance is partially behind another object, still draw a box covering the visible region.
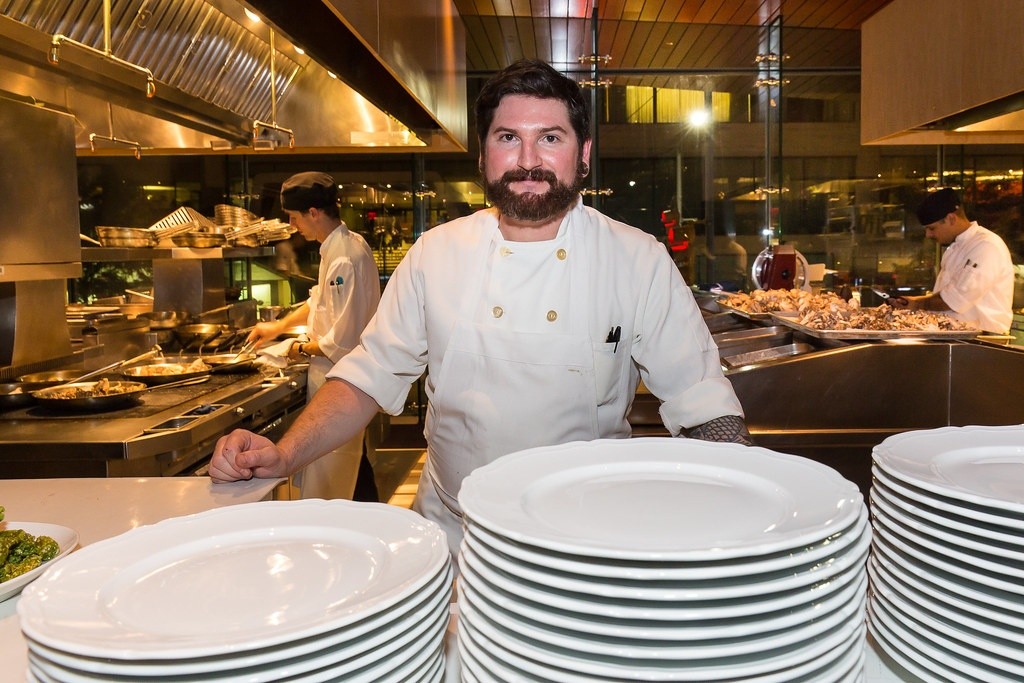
[0,477,290,683]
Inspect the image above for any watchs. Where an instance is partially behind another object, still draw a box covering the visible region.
[299,343,309,357]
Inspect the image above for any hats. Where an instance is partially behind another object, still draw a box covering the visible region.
[914,188,960,226]
[280,171,338,212]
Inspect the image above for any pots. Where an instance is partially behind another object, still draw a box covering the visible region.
[1,315,299,422]
[94,204,298,247]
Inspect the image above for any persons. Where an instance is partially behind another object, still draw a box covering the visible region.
[208,58,751,578]
[248,172,380,502]
[887,190,1014,334]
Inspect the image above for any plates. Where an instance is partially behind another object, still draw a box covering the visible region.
[1,418,1024,682]
